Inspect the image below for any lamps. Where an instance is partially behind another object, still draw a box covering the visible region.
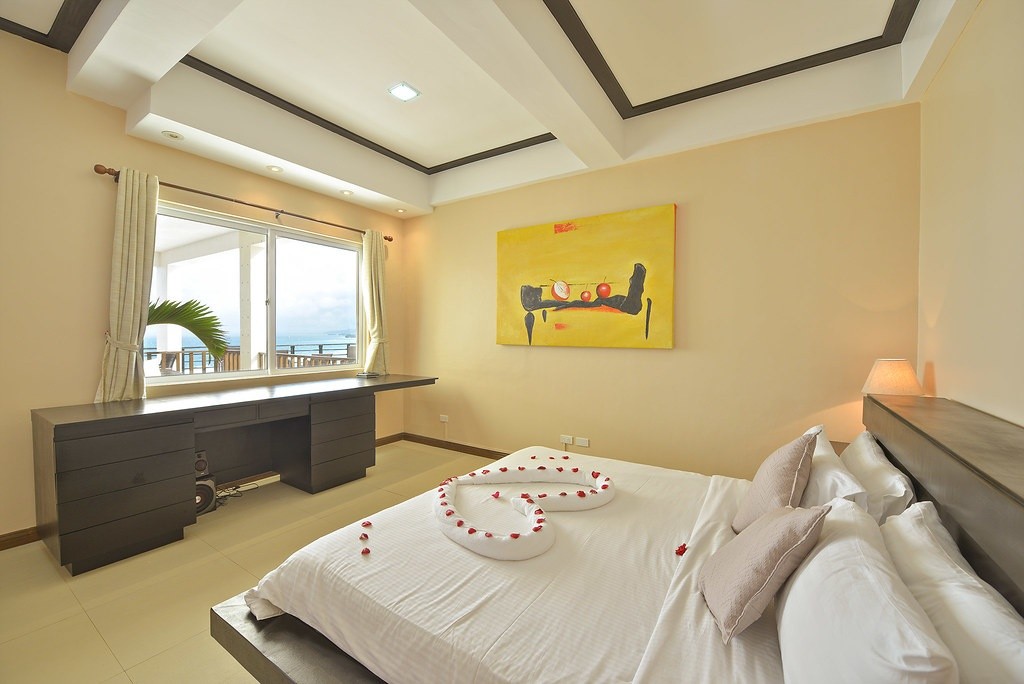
[386,81,422,104]
[861,358,925,397]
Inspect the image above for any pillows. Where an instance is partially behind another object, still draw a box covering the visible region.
[731,429,823,535]
[839,430,918,527]
[879,501,1024,684]
[696,505,832,645]
[798,424,867,512]
[773,496,959,684]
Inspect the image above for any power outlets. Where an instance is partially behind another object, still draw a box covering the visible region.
[560,435,573,444]
[575,437,589,446]
[439,415,449,422]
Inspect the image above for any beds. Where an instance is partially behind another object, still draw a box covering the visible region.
[244,445,785,683]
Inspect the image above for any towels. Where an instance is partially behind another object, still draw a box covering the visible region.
[437,466,618,562]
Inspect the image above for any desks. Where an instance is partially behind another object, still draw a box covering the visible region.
[30,373,438,577]
